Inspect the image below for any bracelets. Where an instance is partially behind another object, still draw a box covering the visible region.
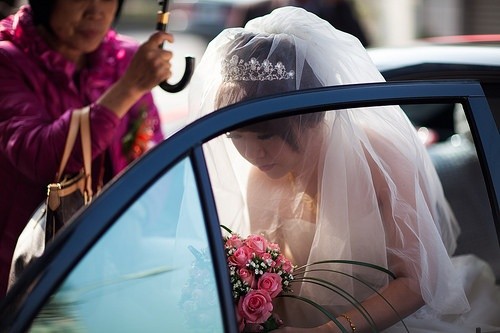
[340,313,356,333]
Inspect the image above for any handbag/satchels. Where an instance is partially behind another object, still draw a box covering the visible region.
[7,104,94,292]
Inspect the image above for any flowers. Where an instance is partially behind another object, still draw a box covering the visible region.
[120,106,152,161]
[179,226,410,333]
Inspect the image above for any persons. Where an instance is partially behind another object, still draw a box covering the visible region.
[0,0,174,297]
[174,6,500,333]
[242,0,371,50]
